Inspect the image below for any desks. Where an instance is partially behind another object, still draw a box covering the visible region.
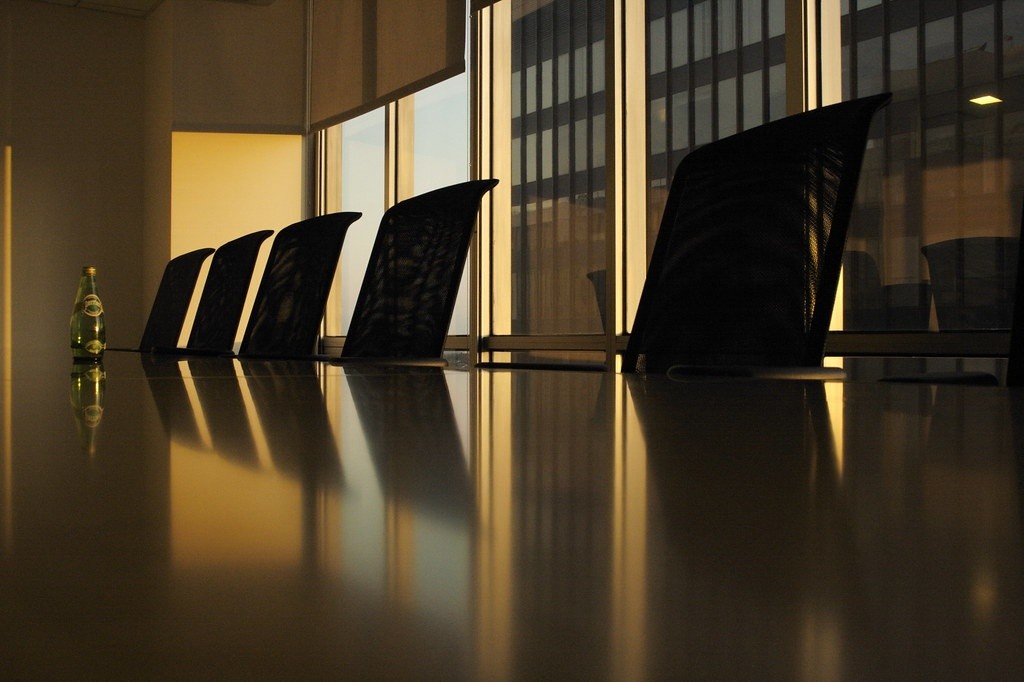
[0,352,1024,682]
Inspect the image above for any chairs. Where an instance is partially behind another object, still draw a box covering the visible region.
[106,247,216,352]
[310,179,499,366]
[843,236,1018,330]
[586,269,607,334]
[237,211,362,357]
[151,230,275,354]
[478,93,894,377]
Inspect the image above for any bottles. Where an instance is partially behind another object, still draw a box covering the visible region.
[69,266,106,362]
[69,362,106,457]
